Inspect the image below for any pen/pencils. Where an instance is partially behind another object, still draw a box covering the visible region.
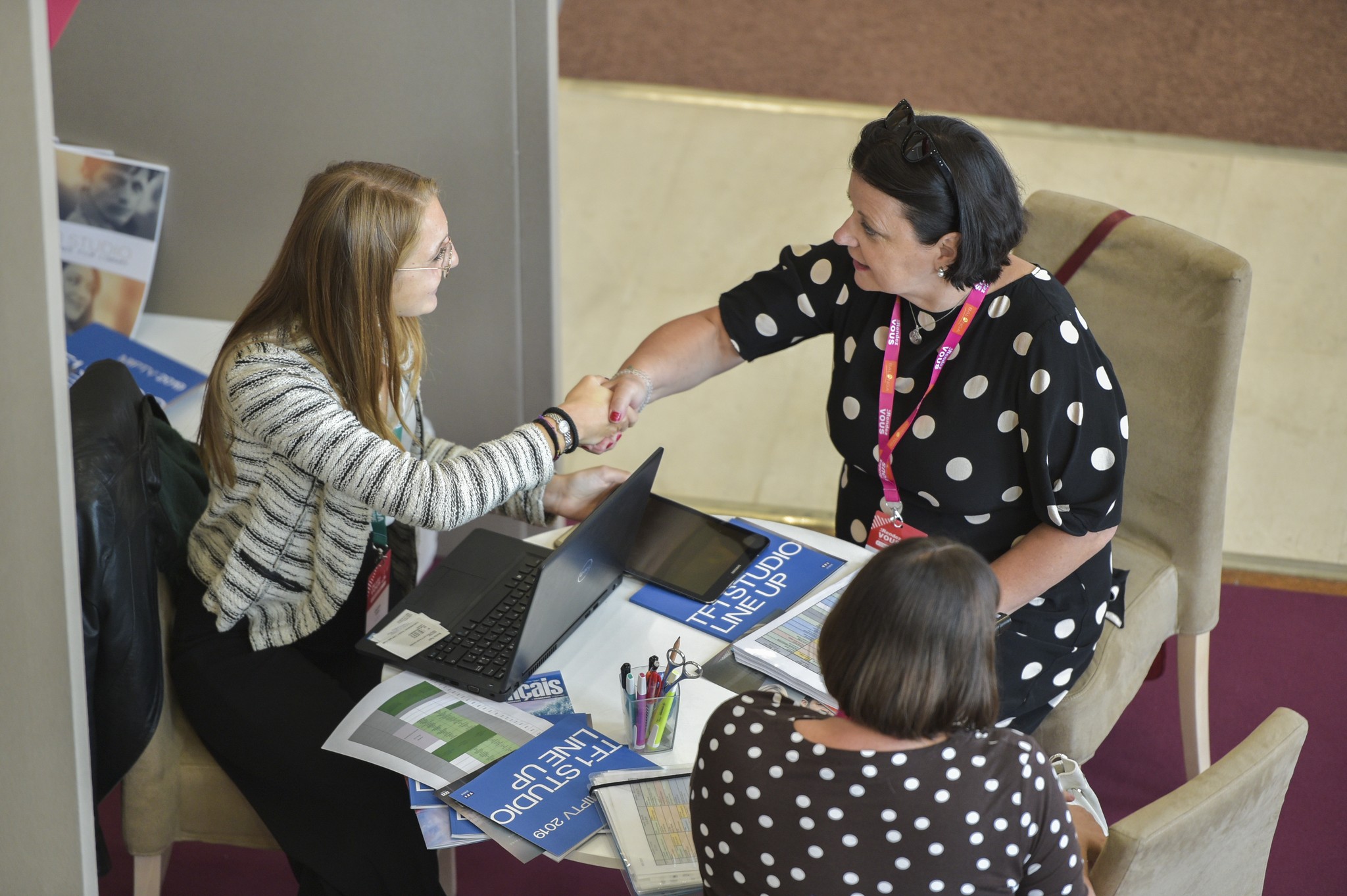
[620,662,631,691]
[657,636,680,688]
[648,655,659,670]
[649,660,660,672]
[645,671,652,680]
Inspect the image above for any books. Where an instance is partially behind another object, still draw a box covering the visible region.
[408,670,703,895]
[629,517,845,643]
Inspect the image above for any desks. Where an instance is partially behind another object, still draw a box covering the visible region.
[381,516,879,896]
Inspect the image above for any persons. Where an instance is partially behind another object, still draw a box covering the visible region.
[687,539,1109,896]
[60,158,156,233]
[61,260,99,344]
[604,113,1131,743]
[170,158,638,895]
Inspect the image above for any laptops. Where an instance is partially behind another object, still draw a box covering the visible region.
[350,446,665,703]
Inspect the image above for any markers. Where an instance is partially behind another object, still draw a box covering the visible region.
[647,669,680,750]
[632,672,648,752]
[625,672,637,735]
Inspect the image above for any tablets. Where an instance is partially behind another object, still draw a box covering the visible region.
[554,483,771,603]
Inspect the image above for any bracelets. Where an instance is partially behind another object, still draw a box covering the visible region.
[611,368,651,413]
[532,418,561,462]
[542,407,579,454]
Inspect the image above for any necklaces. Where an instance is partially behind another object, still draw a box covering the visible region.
[909,288,971,347]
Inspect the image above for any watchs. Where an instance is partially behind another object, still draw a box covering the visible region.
[542,412,572,454]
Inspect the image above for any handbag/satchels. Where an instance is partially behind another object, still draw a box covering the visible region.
[1049,753,1109,837]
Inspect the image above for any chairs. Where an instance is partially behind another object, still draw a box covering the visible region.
[1089,706,1308,896]
[69,360,284,896]
[1011,190,1251,782]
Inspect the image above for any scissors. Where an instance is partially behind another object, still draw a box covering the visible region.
[655,648,704,709]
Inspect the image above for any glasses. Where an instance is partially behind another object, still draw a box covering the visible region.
[394,221,452,279]
[885,98,957,200]
